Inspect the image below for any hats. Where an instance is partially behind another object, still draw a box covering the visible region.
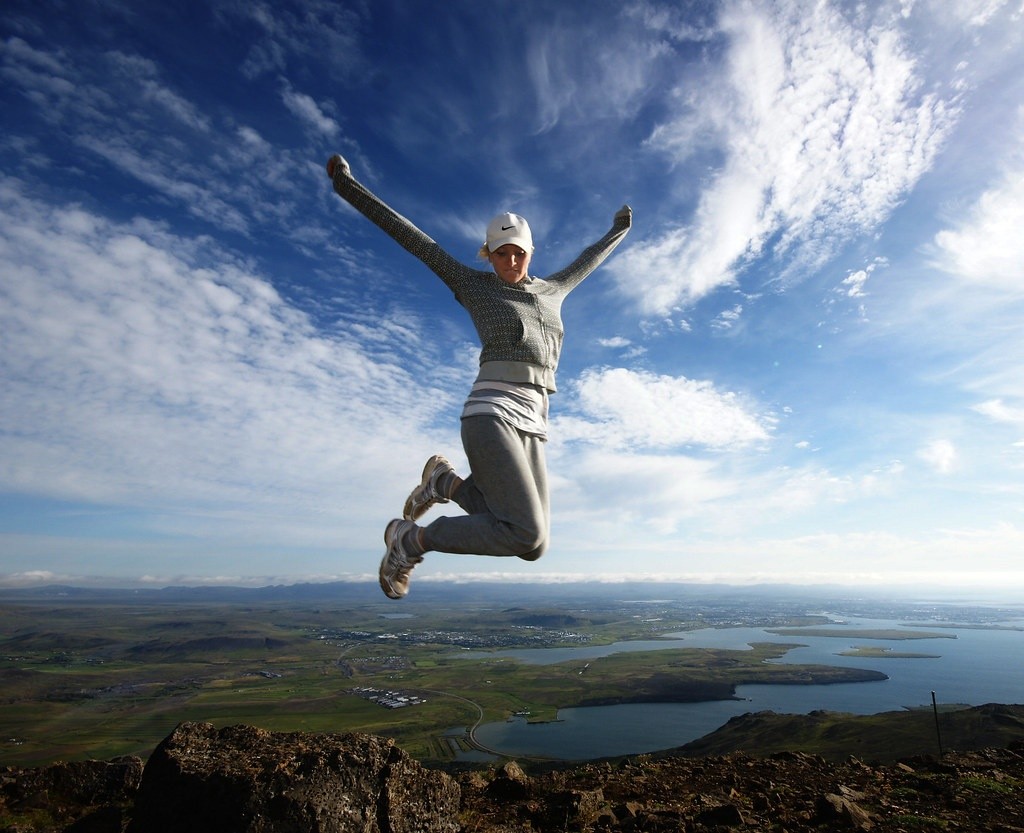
[487,211,534,253]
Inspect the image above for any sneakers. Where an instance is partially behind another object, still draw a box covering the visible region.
[403,454,455,522]
[378,518,425,600]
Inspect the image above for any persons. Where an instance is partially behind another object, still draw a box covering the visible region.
[326,151,634,600]
